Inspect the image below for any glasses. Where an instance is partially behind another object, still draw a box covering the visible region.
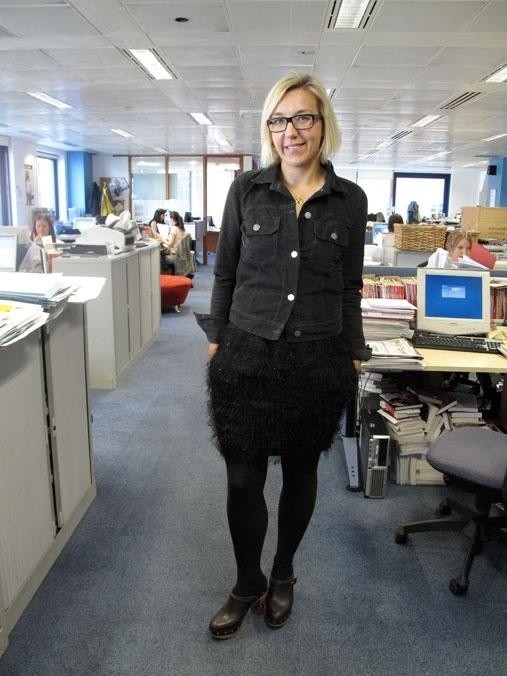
[266,113,321,133]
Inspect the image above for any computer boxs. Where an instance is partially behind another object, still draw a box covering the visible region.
[360,410,390,499]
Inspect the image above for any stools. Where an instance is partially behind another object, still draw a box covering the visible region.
[395,426,507,595]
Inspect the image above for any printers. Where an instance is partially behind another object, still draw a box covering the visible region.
[85,224,140,249]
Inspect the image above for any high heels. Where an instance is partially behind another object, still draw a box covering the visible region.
[265,565,297,627]
[209,576,268,639]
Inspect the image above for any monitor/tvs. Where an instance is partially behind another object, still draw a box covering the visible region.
[73,217,96,233]
[416,268,491,337]
[0,225,29,244]
[372,222,390,244]
[0,234,18,272]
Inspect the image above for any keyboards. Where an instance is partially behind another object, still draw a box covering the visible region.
[413,335,504,354]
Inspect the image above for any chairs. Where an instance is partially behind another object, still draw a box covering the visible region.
[166,234,191,277]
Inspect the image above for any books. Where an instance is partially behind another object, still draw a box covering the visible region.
[360,270,507,486]
[0,272,106,346]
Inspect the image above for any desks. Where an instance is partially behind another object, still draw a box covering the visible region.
[344,345,507,493]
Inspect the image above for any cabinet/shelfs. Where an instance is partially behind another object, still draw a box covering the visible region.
[51,241,162,389]
[0,304,97,661]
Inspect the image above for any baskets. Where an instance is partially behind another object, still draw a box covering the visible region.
[394,223,447,251]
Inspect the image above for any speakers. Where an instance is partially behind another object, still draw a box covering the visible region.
[487,165,496,175]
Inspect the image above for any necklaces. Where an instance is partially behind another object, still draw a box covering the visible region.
[288,185,318,203]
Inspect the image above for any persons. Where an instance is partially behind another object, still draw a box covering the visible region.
[425,228,473,269]
[149,207,167,235]
[366,199,420,233]
[30,215,65,243]
[192,67,375,638]
[162,210,185,272]
